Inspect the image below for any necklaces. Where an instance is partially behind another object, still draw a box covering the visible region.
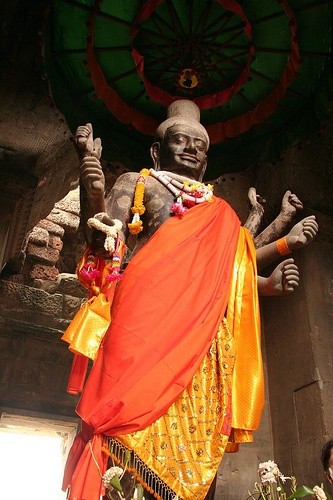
[129,168,215,236]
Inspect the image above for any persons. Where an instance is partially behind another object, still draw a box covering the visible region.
[59,99,317,500]
[321,439,333,487]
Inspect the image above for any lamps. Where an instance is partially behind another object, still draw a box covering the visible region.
[178,69,199,88]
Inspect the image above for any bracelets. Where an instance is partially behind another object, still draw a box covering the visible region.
[276,237,294,257]
